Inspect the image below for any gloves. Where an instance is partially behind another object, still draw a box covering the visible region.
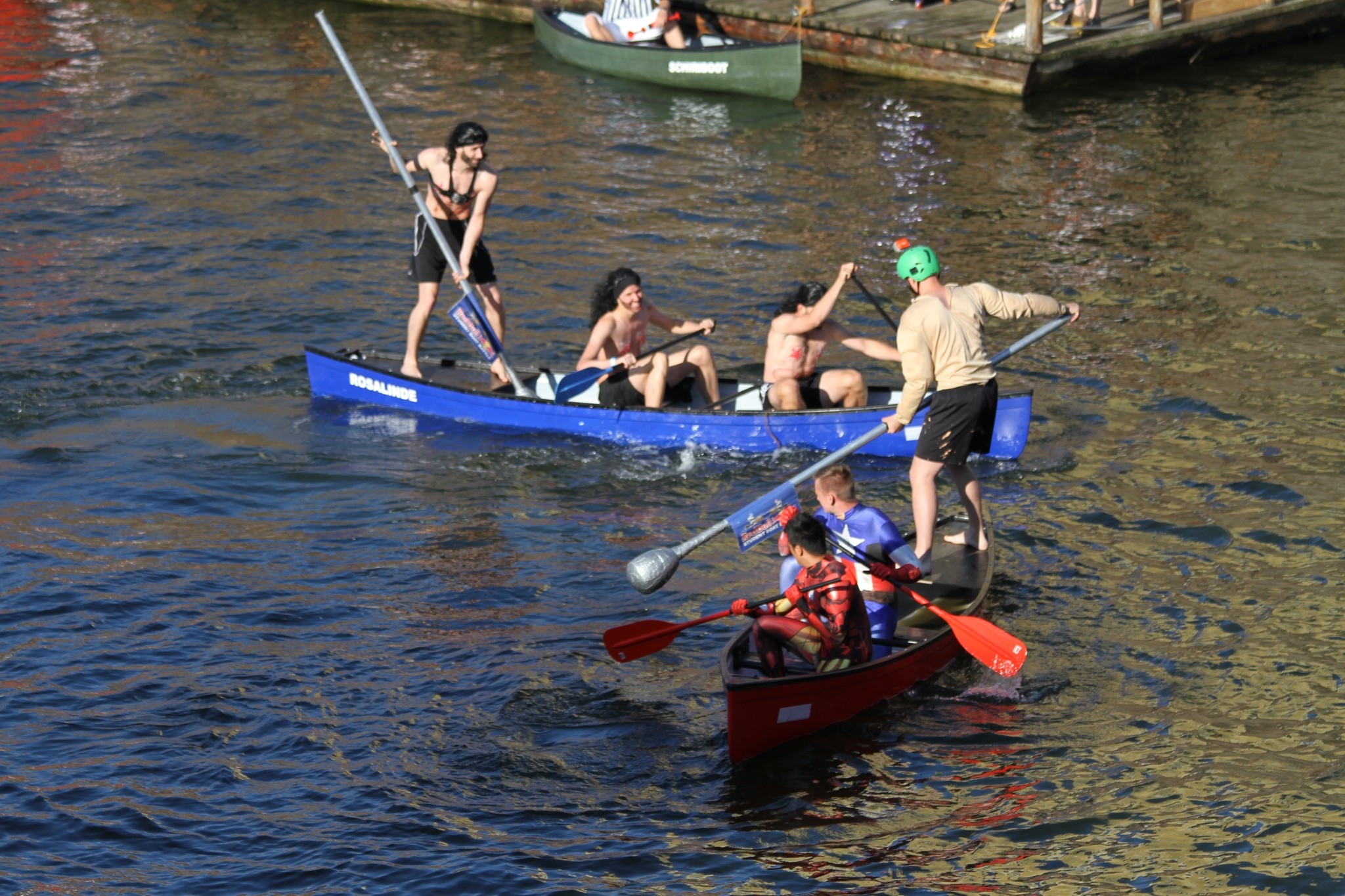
[778,506,800,555]
[871,563,921,585]
[731,599,753,617]
[785,585,805,606]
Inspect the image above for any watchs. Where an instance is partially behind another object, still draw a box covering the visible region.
[660,7,669,13]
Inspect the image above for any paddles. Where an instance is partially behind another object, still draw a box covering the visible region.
[849,268,898,332]
[602,567,859,665]
[825,535,1029,681]
[553,320,717,406]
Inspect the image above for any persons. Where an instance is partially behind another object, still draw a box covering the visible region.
[761,263,900,409]
[731,511,873,674]
[778,462,932,662]
[371,121,511,384]
[583,0,687,50]
[881,245,1081,574]
[576,266,724,410]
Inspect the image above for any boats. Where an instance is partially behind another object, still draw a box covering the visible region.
[716,498,995,769]
[303,338,1035,463]
[531,5,804,103]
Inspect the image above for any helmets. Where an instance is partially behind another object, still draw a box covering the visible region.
[896,245,941,281]
